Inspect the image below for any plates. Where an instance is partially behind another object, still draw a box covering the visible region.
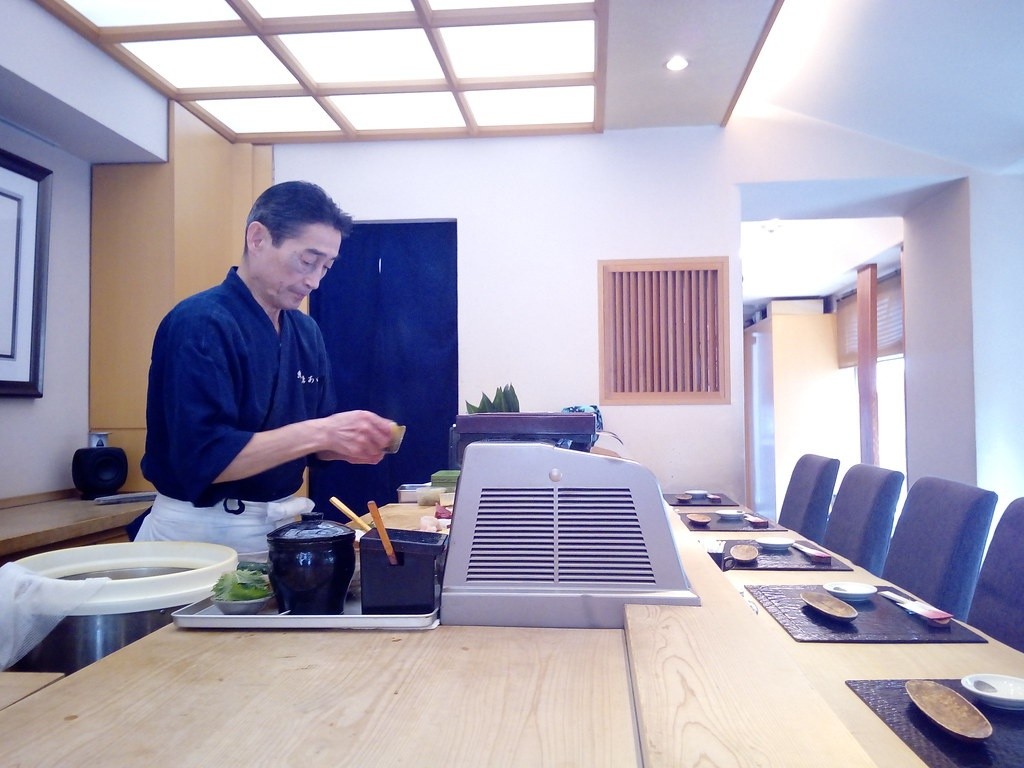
[687,514,711,525]
[905,681,993,742]
[716,510,744,520]
[730,545,759,563]
[685,490,707,499]
[822,583,878,601]
[756,537,794,550]
[675,495,692,500]
[800,592,858,622]
[961,674,1024,710]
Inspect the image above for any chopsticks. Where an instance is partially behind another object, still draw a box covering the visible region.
[329,496,370,531]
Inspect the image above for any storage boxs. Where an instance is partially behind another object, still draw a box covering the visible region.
[430,470,460,487]
[416,487,447,506]
[767,299,824,317]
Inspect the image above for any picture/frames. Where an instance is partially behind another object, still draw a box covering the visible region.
[0,148,54,398]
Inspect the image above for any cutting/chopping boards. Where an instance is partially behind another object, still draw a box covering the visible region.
[345,502,452,538]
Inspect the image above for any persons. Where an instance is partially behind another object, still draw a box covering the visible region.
[129,180,399,578]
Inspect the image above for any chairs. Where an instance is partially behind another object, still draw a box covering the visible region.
[966,496,1024,653]
[821,464,905,580]
[778,454,840,547]
[881,477,998,624]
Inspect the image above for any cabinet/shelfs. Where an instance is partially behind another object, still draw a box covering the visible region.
[90,100,235,492]
[744,314,838,524]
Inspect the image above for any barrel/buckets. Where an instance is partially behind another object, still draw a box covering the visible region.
[0,542,239,673]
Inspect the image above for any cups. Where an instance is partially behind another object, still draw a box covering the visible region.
[266,512,357,616]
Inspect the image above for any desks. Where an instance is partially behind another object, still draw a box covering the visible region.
[0,497,156,566]
[622,492,1024,768]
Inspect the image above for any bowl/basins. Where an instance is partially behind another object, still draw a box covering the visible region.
[210,595,269,614]
